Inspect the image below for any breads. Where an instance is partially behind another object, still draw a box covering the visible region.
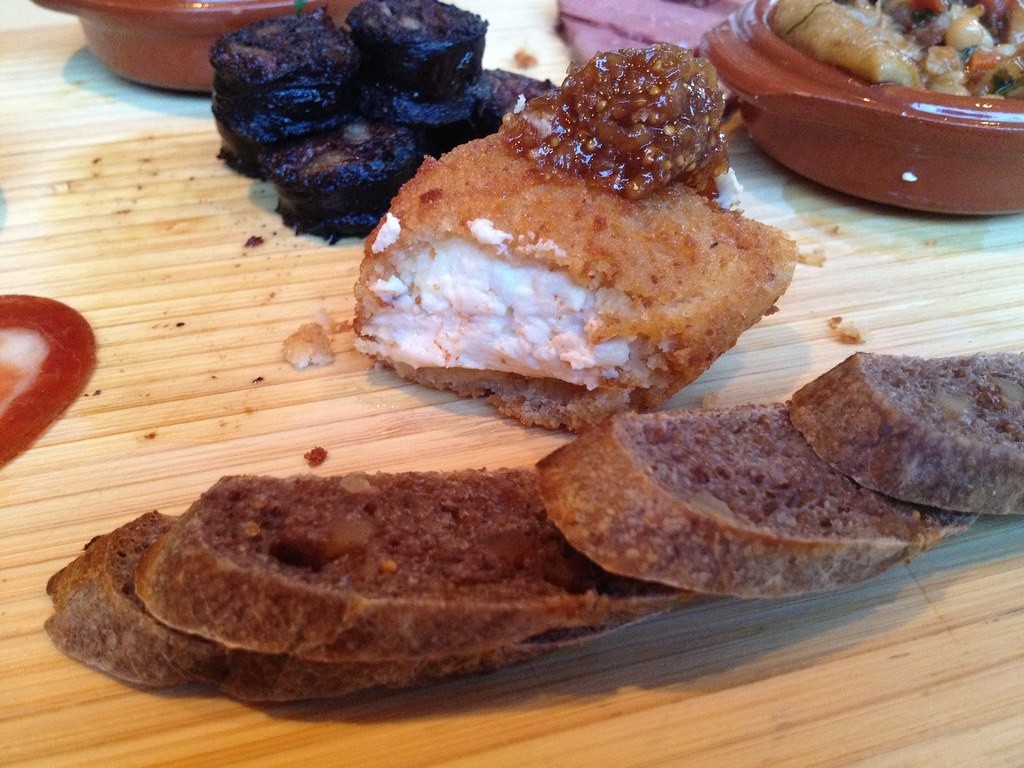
[39,346,1022,707]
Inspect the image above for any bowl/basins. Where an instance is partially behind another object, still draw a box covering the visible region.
[35,1,364,94]
[698,1,1023,218]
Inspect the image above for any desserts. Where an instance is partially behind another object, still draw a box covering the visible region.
[350,43,804,432]
